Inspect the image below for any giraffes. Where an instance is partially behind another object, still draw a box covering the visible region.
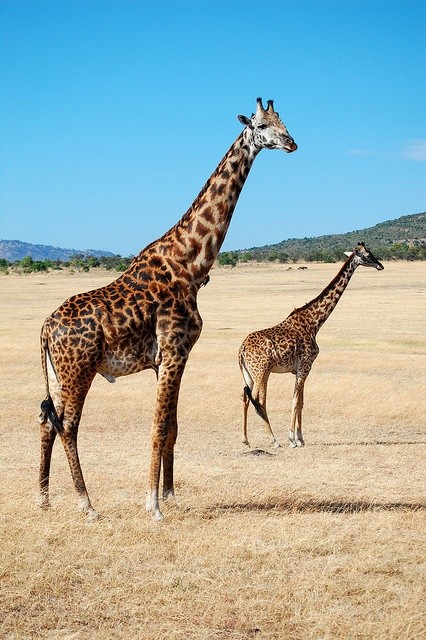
[236,240,386,452]
[35,96,299,523]
